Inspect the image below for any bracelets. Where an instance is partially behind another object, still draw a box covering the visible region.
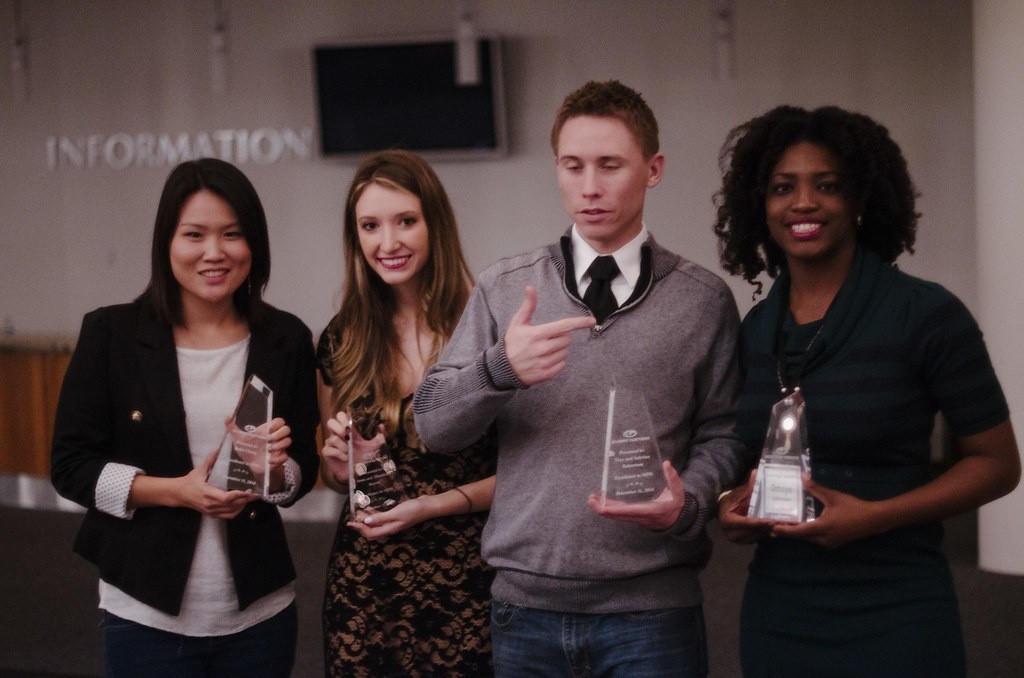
[453,487,473,517]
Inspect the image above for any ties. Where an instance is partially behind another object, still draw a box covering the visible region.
[583,256,619,325]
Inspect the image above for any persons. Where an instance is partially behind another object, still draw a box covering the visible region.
[413,77,750,678]
[50,157,321,678]
[316,150,498,678]
[710,104,1021,678]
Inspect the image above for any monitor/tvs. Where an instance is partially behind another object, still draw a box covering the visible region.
[311,36,498,156]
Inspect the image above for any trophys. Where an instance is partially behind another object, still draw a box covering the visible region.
[347,397,411,526]
[746,392,817,525]
[601,388,675,506]
[205,374,272,502]
